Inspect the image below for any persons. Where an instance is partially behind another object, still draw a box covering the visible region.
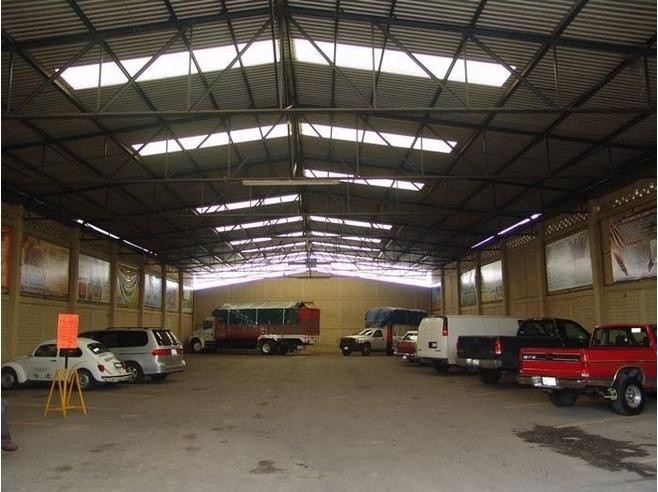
[2,406,18,450]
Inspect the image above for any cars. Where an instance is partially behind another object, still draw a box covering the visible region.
[2,327,186,390]
[393,330,417,362]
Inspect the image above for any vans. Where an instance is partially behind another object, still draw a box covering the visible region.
[417,315,526,373]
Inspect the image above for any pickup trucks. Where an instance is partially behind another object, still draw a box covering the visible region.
[339,328,403,356]
[455,316,656,416]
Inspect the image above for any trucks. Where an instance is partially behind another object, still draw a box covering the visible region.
[188,300,320,355]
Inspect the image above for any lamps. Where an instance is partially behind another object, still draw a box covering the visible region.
[240,179,341,186]
